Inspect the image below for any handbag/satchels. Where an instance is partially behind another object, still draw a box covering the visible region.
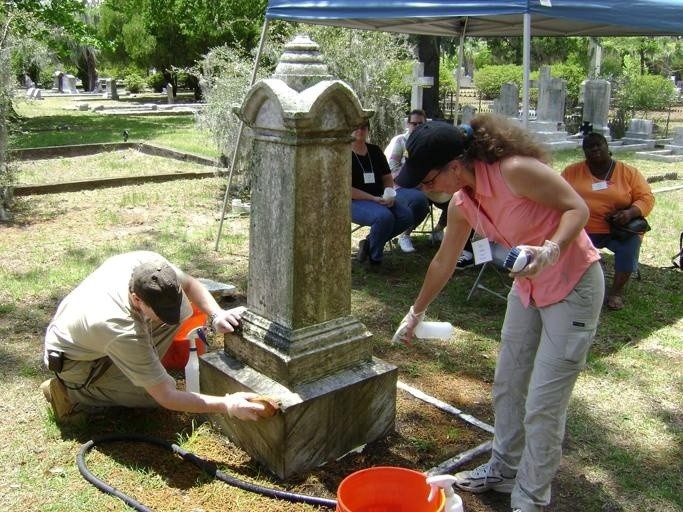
[604,206,652,242]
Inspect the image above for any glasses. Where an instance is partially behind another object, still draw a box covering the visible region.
[420,154,464,189]
[409,121,423,127]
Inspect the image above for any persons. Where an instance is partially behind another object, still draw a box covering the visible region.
[40,250,265,425]
[382,110,450,255]
[391,116,604,511]
[351,116,413,265]
[559,132,656,310]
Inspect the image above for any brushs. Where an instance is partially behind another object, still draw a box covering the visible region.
[503,246,531,273]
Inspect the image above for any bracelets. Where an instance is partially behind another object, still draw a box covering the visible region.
[209,312,217,321]
[224,392,235,421]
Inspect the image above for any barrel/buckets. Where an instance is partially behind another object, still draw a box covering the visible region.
[335,464,447,512]
[161,300,206,369]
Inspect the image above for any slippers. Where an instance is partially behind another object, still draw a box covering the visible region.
[607,295,624,310]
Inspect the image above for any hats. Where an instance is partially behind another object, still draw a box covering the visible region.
[393,121,461,190]
[134,262,182,324]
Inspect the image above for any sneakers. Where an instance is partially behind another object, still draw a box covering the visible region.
[357,225,476,271]
[40,377,87,427]
[454,463,516,493]
